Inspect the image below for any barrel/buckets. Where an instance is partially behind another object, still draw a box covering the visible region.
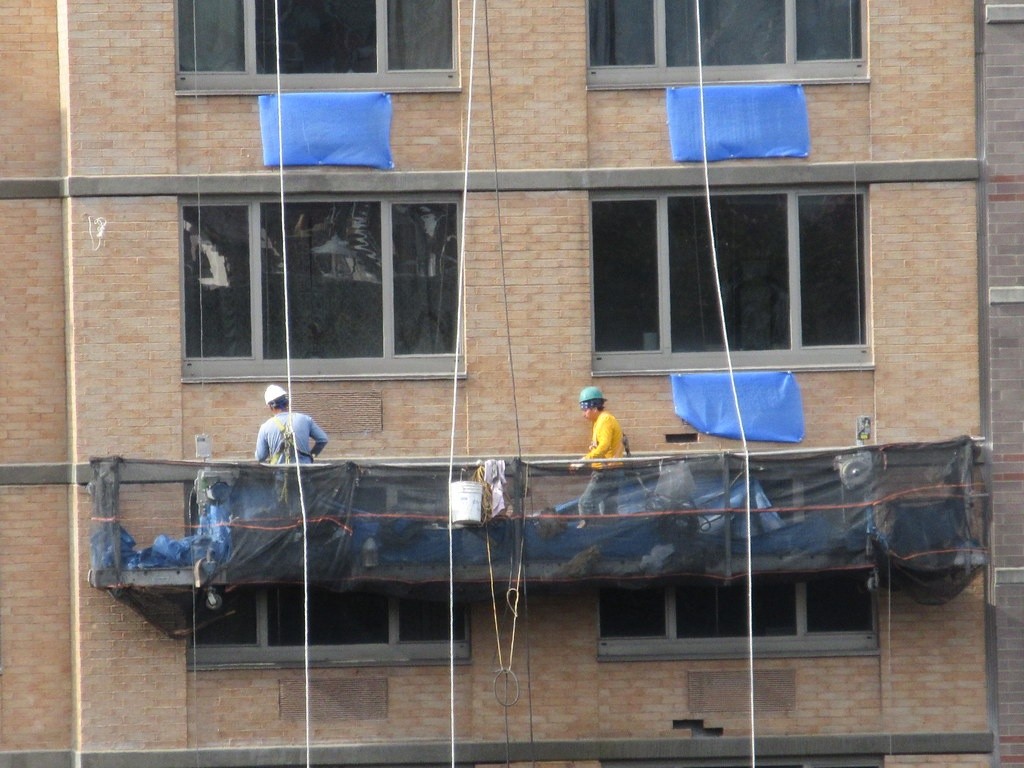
[450,469,483,525]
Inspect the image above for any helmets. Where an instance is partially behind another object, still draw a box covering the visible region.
[265,385,287,404]
[579,386,608,404]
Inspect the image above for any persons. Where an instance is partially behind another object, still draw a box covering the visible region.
[254,384,328,498]
[569,385,624,520]
